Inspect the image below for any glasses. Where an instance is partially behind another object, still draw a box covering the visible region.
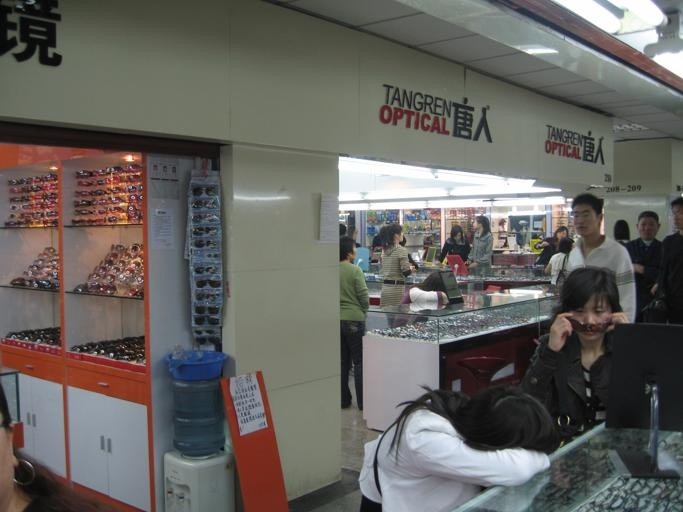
[195,240,216,249]
[10,183,57,193]
[73,204,143,215]
[79,173,142,186]
[196,293,218,303]
[198,337,216,344]
[10,202,57,210]
[8,211,58,220]
[196,280,221,288]
[74,244,144,297]
[191,199,217,208]
[73,186,142,197]
[8,174,57,184]
[70,216,143,224]
[72,194,143,207]
[7,328,60,346]
[10,193,58,203]
[192,227,217,236]
[75,163,142,179]
[195,267,217,274]
[194,317,219,325]
[195,306,220,313]
[193,187,218,196]
[5,219,58,227]
[464,421,682,512]
[192,214,217,223]
[11,247,59,288]
[72,336,144,363]
[195,330,214,335]
[568,318,611,332]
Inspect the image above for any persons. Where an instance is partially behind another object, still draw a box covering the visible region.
[564,192,638,326]
[339,235,370,411]
[0,381,98,511]
[336,210,578,317]
[622,210,667,326]
[610,218,633,247]
[652,196,683,325]
[515,264,633,433]
[358,387,571,510]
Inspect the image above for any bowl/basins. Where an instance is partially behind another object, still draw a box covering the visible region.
[164,350,228,381]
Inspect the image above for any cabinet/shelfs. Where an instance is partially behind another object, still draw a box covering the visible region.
[340,207,488,250]
[359,263,554,306]
[0,344,68,492]
[363,285,565,429]
[65,359,176,512]
[0,151,215,373]
[449,420,683,511]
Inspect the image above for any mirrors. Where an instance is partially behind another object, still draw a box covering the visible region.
[606,320,683,479]
[424,247,438,266]
[445,254,469,276]
[437,270,464,304]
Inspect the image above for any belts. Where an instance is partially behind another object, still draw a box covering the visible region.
[383,279,405,285]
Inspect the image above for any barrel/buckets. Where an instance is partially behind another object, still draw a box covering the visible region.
[170,379,226,457]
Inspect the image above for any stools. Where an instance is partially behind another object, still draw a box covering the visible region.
[456,357,521,393]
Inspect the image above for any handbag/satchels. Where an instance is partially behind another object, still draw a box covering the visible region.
[555,268,567,296]
